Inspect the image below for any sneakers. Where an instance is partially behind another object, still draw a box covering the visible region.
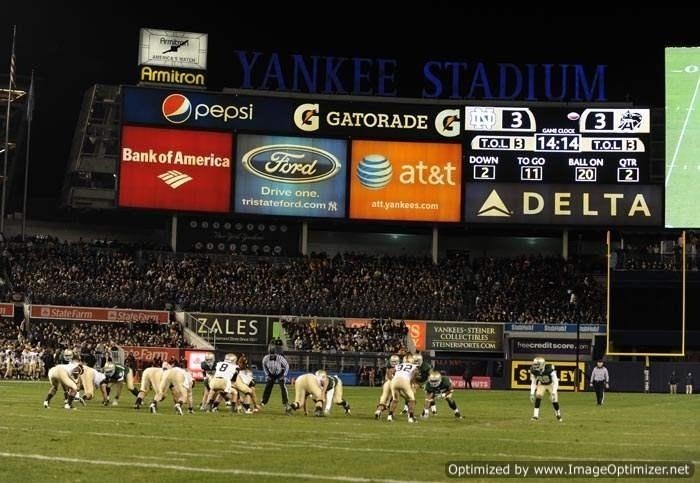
[374,410,461,423]
[134,397,183,415]
[344,408,351,417]
[285,405,323,417]
[44,397,119,410]
[200,402,264,414]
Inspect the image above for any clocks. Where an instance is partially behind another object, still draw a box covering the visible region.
[136,27,209,72]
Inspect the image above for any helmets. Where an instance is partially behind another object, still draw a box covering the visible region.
[205,352,249,368]
[390,353,423,367]
[103,361,115,377]
[268,343,277,355]
[427,371,442,388]
[532,357,545,375]
[316,370,327,380]
[63,348,74,362]
